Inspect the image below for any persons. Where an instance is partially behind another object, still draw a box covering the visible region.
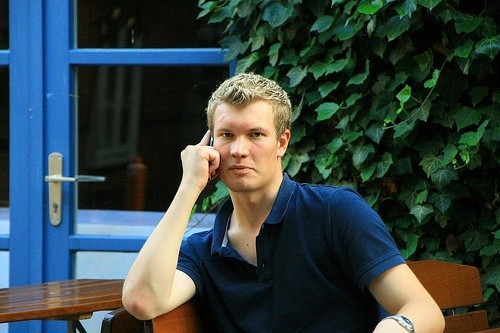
[121,72,445,333]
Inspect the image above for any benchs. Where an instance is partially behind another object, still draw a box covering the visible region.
[139,256,500,333]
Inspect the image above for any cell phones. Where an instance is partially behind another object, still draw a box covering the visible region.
[208,135,214,182]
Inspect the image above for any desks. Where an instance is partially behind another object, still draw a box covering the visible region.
[1,277,127,333]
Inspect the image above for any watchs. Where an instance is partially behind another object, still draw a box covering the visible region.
[384,315,414,333]
